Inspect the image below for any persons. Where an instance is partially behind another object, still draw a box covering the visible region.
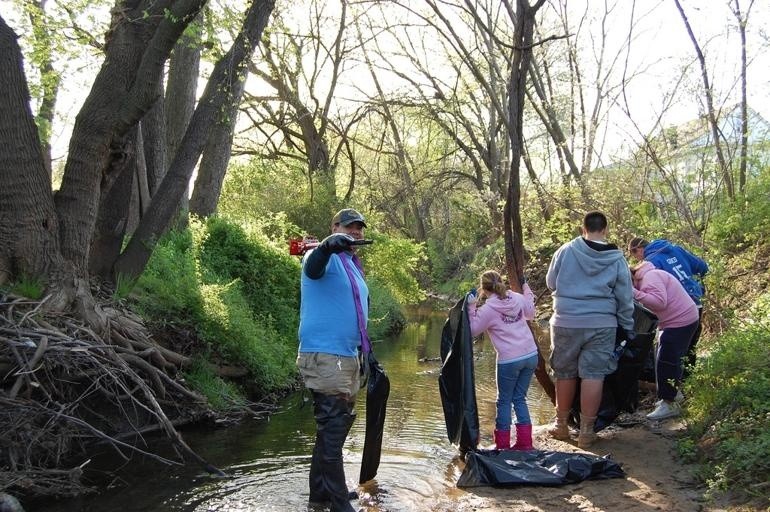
[464,269,540,452]
[628,237,710,383]
[626,260,701,419]
[544,211,635,451]
[297,208,380,511]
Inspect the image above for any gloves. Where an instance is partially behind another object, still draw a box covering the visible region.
[317,233,354,253]
[467,294,479,304]
[366,353,379,375]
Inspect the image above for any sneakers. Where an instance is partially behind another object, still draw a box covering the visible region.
[645,391,684,420]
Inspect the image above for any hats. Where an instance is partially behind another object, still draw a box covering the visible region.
[332,209,368,227]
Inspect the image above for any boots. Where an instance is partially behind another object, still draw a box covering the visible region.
[549,408,569,439]
[578,414,599,448]
[493,429,510,452]
[511,423,535,451]
[309,392,359,511]
